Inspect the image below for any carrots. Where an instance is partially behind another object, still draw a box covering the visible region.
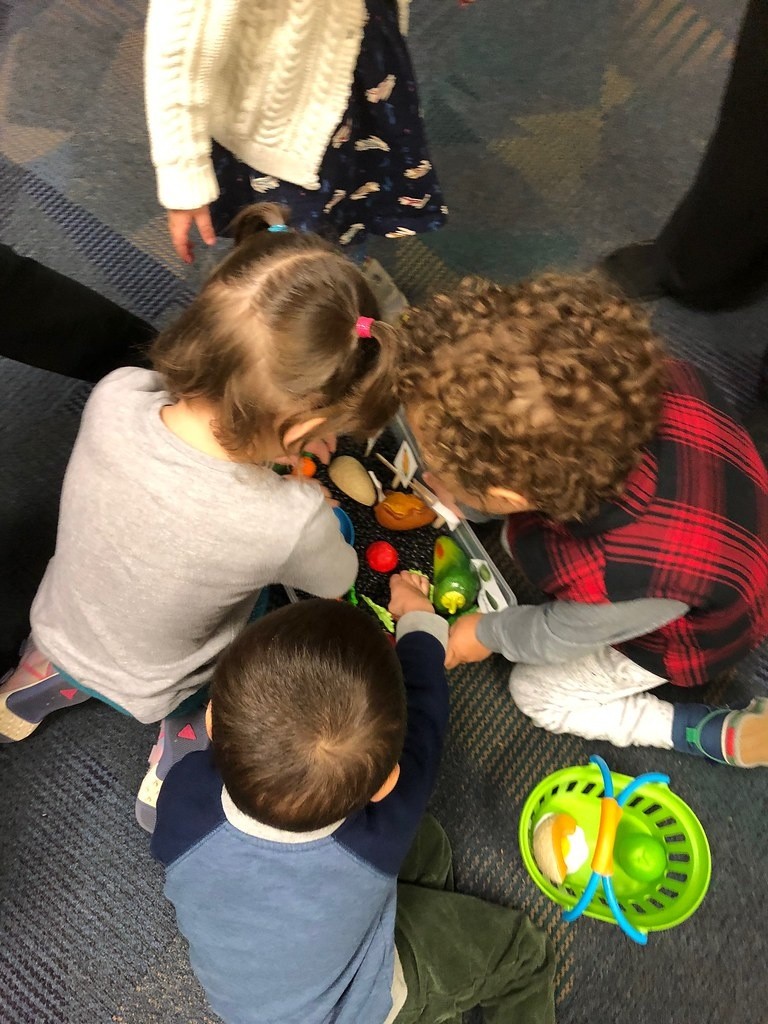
[290,454,317,477]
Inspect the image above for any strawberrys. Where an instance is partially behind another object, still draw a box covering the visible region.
[366,541,398,573]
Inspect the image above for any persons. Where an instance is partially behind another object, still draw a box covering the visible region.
[606,1,768,305]
[1,195,403,844]
[140,0,453,346]
[389,273,768,777]
[140,572,561,1023]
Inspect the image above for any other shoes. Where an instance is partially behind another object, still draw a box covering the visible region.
[598,238,667,301]
[135,696,211,836]
[0,633,92,743]
[685,696,768,770]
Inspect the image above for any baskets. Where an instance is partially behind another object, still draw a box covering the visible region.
[519,755,712,946]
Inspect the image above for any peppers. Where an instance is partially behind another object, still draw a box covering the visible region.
[432,536,478,616]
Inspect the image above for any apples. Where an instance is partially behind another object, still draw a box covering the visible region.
[621,833,666,882]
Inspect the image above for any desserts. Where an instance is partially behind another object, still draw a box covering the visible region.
[534,813,589,885]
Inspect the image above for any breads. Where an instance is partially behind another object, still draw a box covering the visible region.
[373,492,437,531]
[327,456,377,505]
